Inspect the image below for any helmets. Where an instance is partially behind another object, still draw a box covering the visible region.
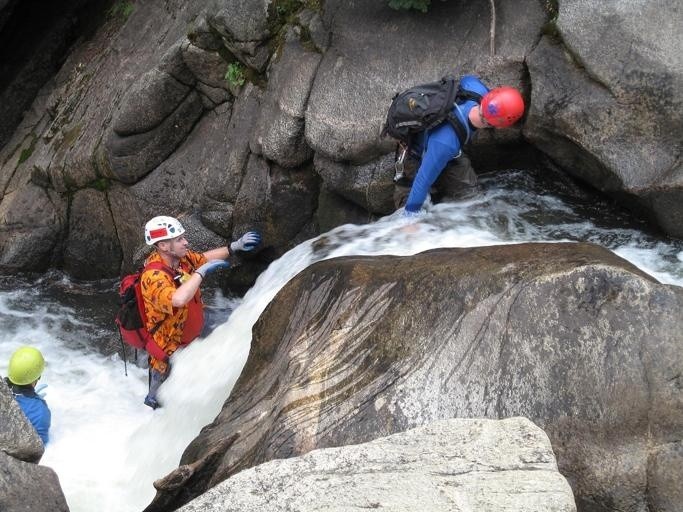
[145,216,186,245]
[481,86,525,127]
[8,347,44,386]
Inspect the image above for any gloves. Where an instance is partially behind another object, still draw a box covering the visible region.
[231,231,260,252]
[194,259,230,278]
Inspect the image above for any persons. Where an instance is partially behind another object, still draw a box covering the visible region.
[388,76,524,233]
[1,347,51,452]
[138,215,262,412]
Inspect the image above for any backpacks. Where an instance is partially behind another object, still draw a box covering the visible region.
[114,261,188,348]
[380,74,483,152]
